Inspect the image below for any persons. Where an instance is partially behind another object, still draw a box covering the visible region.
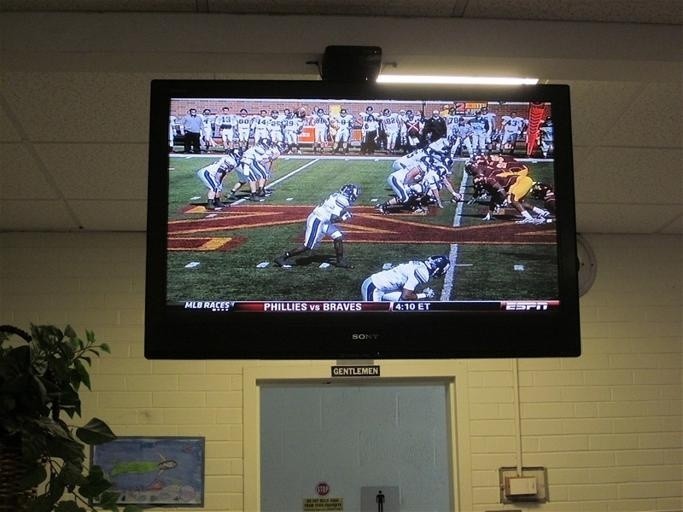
[362,255,451,300]
[169,103,556,267]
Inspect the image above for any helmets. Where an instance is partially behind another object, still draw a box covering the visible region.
[464,160,479,174]
[424,254,450,278]
[228,148,243,161]
[473,176,487,190]
[276,140,285,153]
[260,137,272,150]
[340,183,361,202]
[421,133,458,176]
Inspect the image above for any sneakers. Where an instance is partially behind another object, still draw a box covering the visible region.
[273,257,292,269]
[206,194,238,210]
[336,258,355,269]
[376,201,386,212]
[250,189,273,201]
[515,209,552,225]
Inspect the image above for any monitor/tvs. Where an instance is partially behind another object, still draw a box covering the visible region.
[146,79,582,360]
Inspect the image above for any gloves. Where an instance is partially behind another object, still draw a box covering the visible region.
[468,193,507,220]
[423,286,436,298]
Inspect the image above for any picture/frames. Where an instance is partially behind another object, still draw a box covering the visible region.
[88,436,205,507]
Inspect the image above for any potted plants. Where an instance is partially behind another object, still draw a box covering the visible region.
[0,323,144,512]
